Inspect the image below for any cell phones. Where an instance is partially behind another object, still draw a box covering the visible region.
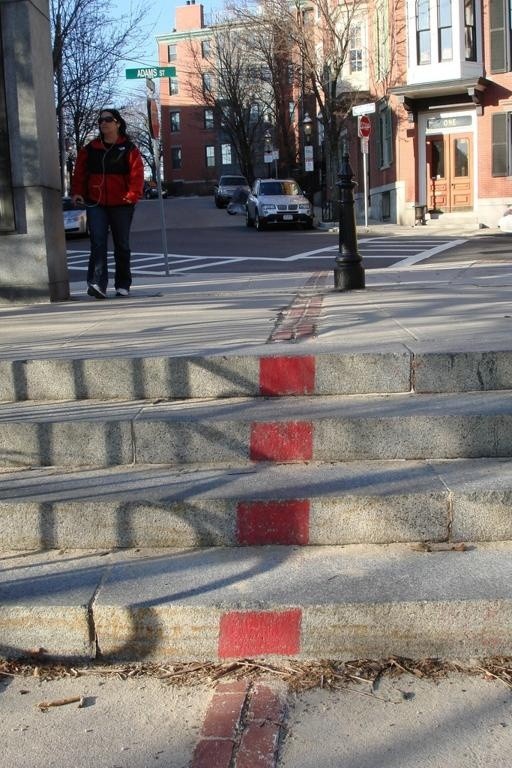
[75,200,88,208]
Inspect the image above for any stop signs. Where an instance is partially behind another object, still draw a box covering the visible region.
[360,116,371,136]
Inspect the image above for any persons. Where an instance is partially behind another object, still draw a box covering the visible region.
[73,109,145,300]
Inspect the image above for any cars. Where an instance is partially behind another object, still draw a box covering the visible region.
[213,175,313,232]
[144,181,168,200]
[62,200,90,238]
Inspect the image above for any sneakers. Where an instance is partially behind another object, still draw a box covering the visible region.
[87,282,107,299]
[115,288,130,297]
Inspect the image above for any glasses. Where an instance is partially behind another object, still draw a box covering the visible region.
[98,116,118,124]
